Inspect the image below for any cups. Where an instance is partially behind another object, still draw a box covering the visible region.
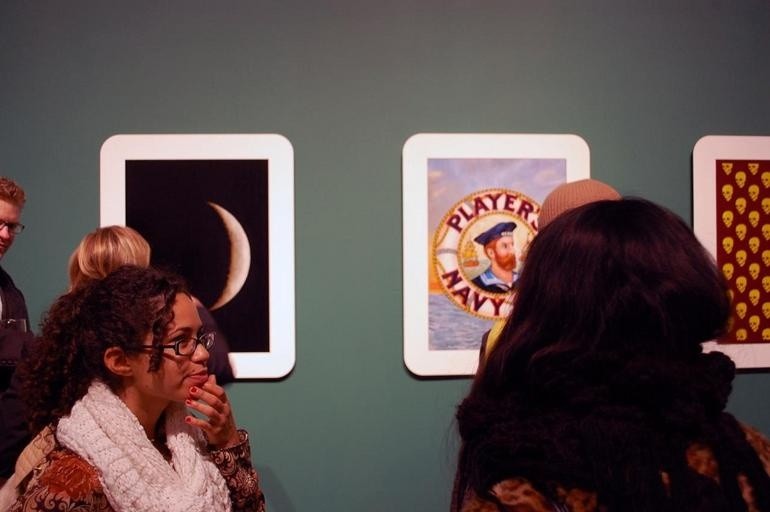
[127,331,219,358]
[0,220,25,234]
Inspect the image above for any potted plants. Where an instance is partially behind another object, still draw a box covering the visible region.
[401,134,593,380]
[97,134,297,386]
[693,137,770,373]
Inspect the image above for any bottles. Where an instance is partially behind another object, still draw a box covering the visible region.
[533,177,622,236]
[474,221,518,245]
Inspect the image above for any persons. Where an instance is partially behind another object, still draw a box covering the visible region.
[471,220,521,295]
[68,227,151,291]
[453,195,770,512]
[0,175,34,478]
[478,181,623,366]
[9,264,266,512]
